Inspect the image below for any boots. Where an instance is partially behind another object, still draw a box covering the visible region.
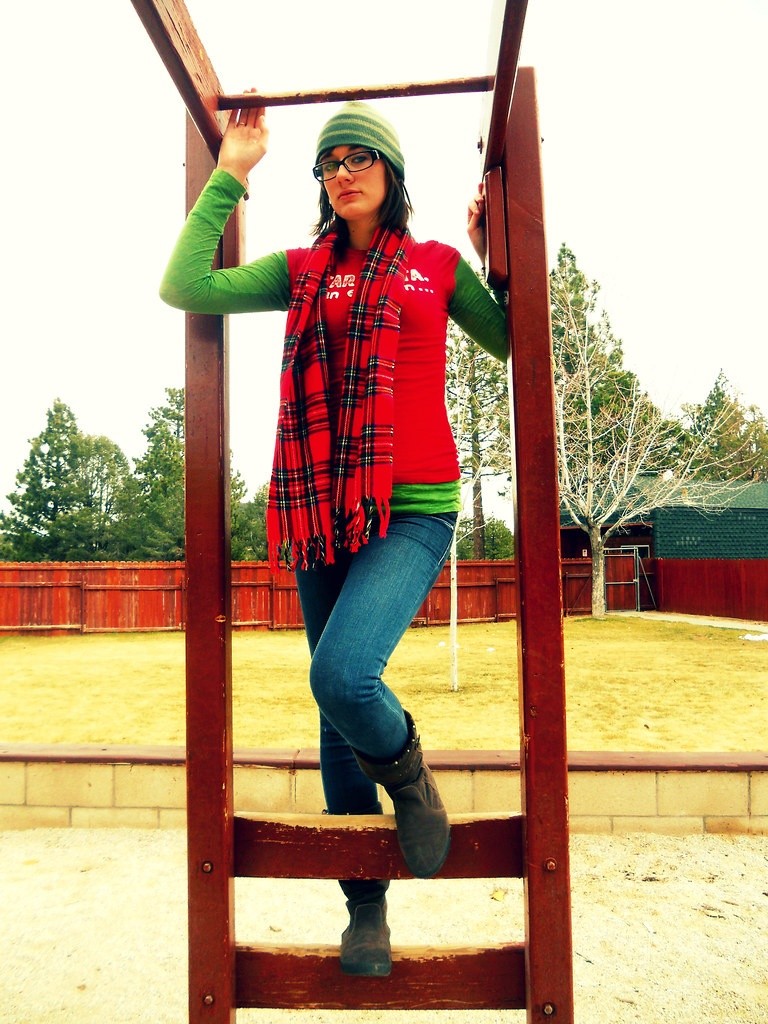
[351,709,450,877]
[322,802,391,977]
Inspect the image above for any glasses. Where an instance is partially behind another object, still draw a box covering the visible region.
[313,150,381,182]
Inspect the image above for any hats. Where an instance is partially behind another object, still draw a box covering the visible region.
[316,101,405,179]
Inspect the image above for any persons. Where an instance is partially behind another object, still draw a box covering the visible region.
[158,87,510,978]
